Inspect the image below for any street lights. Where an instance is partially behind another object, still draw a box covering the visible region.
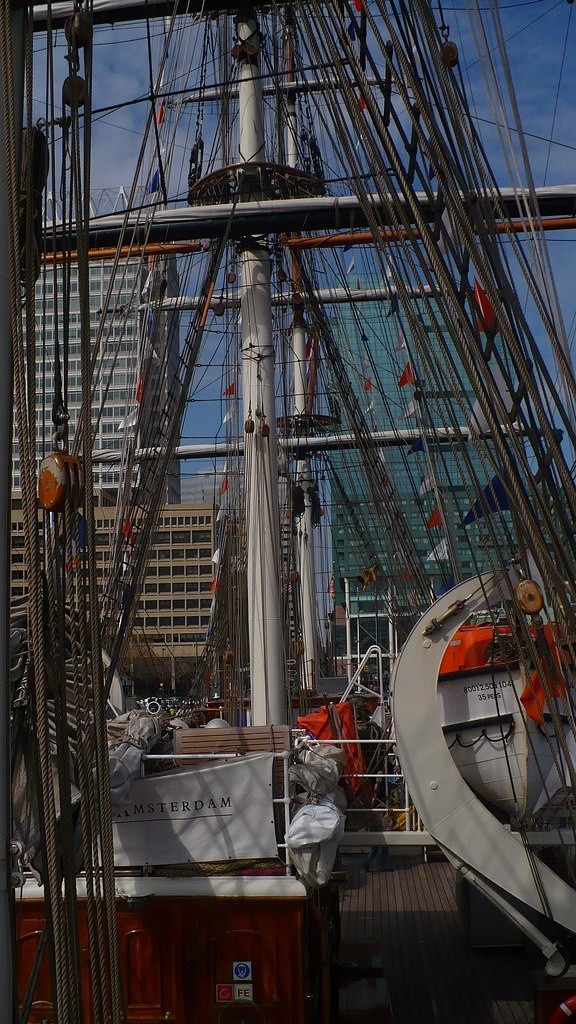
[161,648,166,701]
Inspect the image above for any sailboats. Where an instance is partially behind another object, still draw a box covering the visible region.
[142,0,395,809]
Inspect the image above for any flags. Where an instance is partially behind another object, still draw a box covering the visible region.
[355,565,377,590]
